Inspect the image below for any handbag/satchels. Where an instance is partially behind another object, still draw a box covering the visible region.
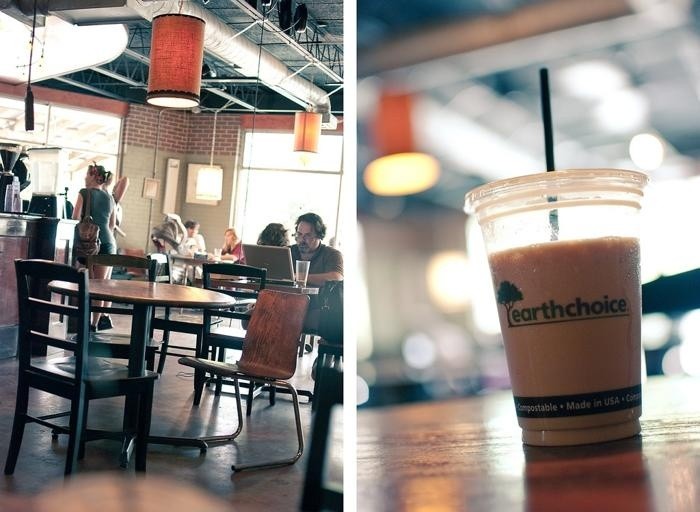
[72,214,102,257]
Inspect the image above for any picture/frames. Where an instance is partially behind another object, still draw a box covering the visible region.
[142,177,162,202]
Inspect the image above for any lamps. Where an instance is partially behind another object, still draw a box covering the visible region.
[291,52,325,157]
[23,2,40,133]
[192,105,224,203]
[144,1,209,114]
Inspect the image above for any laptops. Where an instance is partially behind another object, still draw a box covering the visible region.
[243,244,297,286]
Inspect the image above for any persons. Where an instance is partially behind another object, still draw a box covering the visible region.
[71,164,117,332]
[113,175,130,231]
[170,219,208,288]
[257,223,290,247]
[242,212,343,337]
[207,227,249,291]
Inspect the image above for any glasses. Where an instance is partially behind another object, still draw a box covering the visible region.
[291,232,317,239]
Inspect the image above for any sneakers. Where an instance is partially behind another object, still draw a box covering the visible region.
[89,324,97,333]
[98,315,113,330]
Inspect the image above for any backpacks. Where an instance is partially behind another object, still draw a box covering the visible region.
[315,279,343,345]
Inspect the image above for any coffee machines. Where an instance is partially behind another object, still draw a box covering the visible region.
[0,142,23,213]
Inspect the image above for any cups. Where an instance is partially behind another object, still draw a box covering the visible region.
[214,248,222,262]
[465,168,647,451]
[296,260,311,288]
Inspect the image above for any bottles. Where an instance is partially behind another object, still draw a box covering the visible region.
[4,184,13,213]
[12,176,23,213]
